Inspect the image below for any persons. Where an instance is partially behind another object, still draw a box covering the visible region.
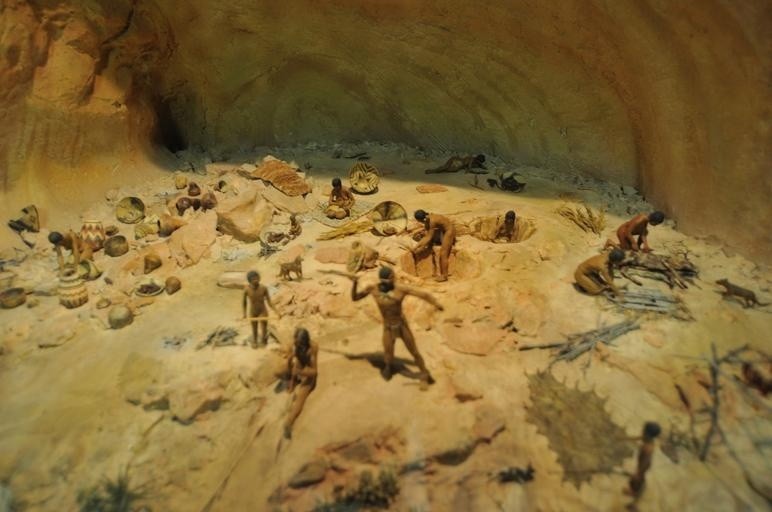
[603,210,665,251]
[411,209,456,283]
[240,270,284,351]
[349,265,444,383]
[270,326,320,440]
[289,215,303,236]
[48,230,96,271]
[620,419,663,497]
[574,247,625,296]
[490,209,523,244]
[325,176,358,219]
[424,154,489,175]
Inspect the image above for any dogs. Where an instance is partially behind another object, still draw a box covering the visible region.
[714,277,772,309]
[276,254,306,281]
